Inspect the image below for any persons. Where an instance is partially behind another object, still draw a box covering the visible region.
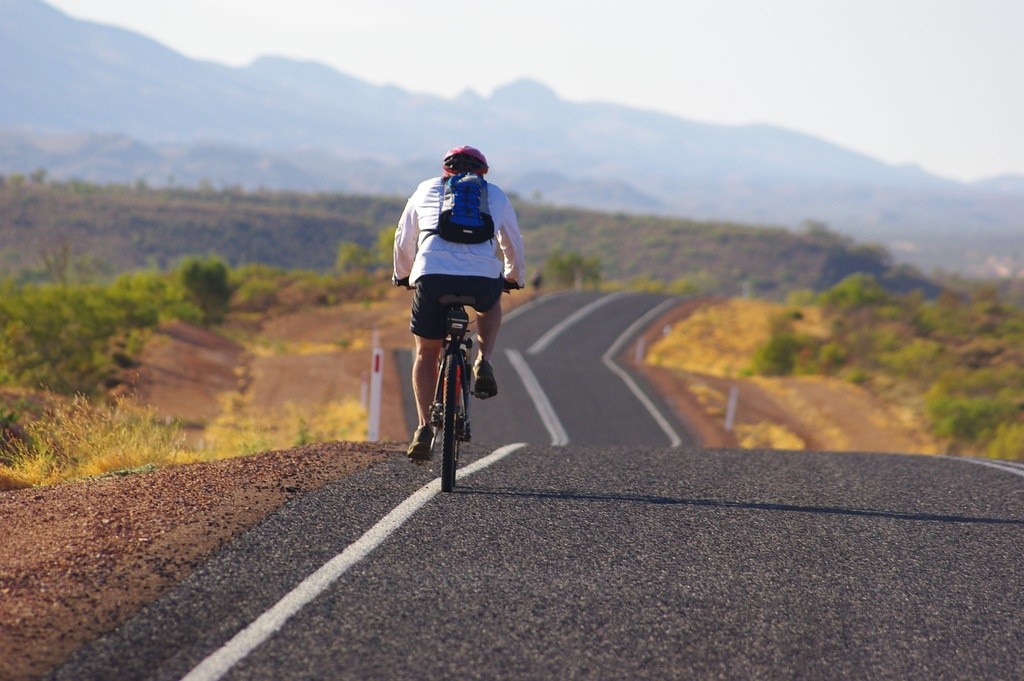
[392,146,525,460]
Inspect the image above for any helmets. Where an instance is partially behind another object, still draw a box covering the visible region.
[442,145,489,174]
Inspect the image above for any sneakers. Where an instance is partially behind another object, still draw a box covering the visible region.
[474,355,497,399]
[407,422,435,460]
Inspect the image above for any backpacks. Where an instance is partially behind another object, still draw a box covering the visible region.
[420,171,495,246]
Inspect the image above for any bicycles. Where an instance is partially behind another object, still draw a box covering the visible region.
[392,277,522,492]
